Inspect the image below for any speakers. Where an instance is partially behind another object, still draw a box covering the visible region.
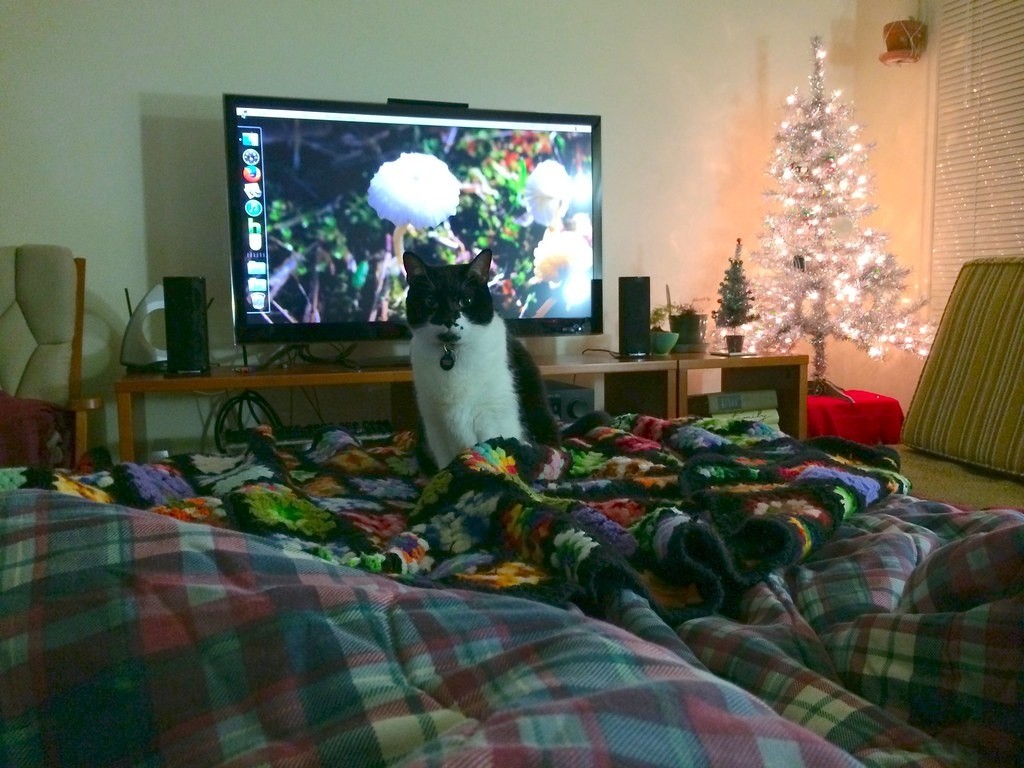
[162,276,210,376]
[542,378,596,421]
[617,276,652,359]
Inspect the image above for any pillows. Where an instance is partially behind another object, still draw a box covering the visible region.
[0,390,73,465]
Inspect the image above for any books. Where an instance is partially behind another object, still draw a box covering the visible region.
[687,388,781,433]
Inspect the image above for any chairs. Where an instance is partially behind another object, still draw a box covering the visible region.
[1,243,104,471]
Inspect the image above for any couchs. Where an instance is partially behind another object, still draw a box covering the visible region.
[0,252,1024,768]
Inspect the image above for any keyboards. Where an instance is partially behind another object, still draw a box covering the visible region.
[224,415,400,452]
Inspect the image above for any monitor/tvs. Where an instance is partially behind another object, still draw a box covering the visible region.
[220,92,602,369]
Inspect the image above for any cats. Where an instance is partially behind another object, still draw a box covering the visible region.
[400,248,617,472]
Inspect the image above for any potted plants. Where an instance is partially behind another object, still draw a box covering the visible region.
[710,238,757,353]
[650,304,710,352]
[879,16,927,66]
[649,308,680,356]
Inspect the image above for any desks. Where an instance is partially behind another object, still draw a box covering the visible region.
[120,353,677,465]
[642,350,810,439]
